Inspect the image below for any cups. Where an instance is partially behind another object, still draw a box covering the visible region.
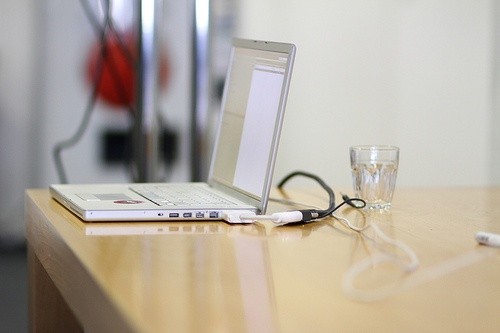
[350,146,400,213]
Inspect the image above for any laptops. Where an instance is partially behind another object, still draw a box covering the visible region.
[49,37,296,221]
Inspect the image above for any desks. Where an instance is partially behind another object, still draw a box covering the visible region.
[24,188,500,333]
